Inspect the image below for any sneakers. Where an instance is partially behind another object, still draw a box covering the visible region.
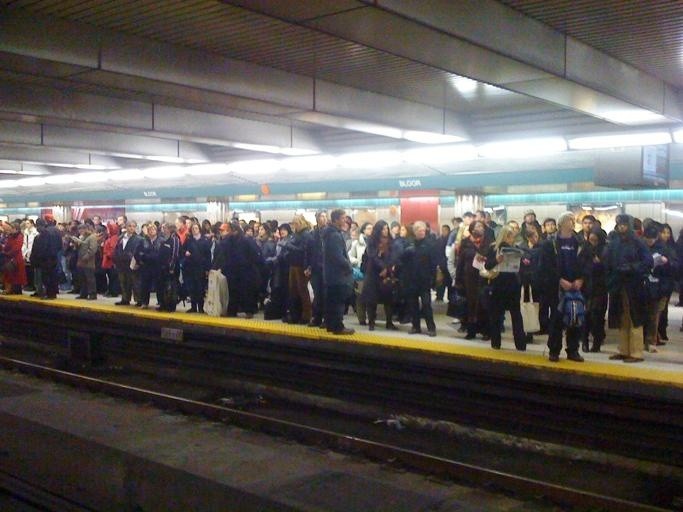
[264,312,355,336]
[97,288,118,297]
[0,289,22,295]
[359,320,437,337]
[451,318,527,351]
[609,353,644,362]
[646,329,669,352]
[565,349,585,362]
[581,338,602,352]
[220,312,237,317]
[187,305,204,313]
[246,311,253,320]
[548,350,560,361]
[115,299,176,311]
[67,292,98,300]
[30,291,60,299]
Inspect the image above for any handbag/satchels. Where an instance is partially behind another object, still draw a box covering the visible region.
[391,270,410,299]
[360,254,384,272]
[562,290,586,328]
[446,295,469,318]
[479,259,500,280]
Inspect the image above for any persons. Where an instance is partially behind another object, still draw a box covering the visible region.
[1,209,358,334]
[341,210,682,363]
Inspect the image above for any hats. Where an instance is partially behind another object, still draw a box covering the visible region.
[644,225,657,238]
[524,209,535,219]
[218,223,230,231]
[614,215,633,232]
